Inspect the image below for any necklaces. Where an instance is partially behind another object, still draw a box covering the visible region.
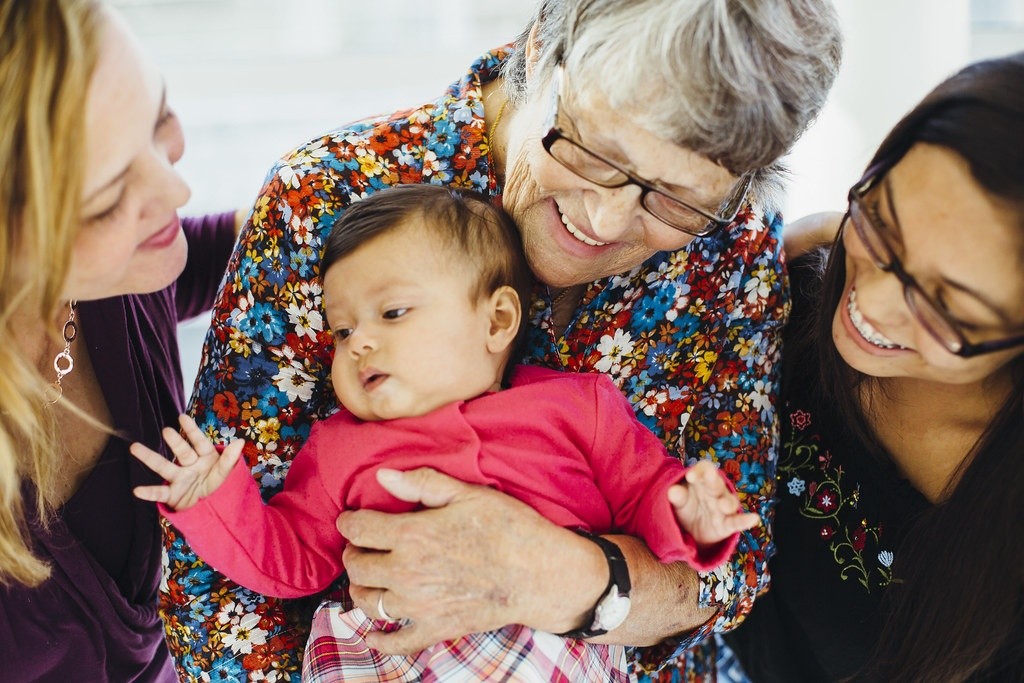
[45,300,78,408]
[489,92,574,308]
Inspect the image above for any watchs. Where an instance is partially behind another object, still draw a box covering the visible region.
[554,530,632,639]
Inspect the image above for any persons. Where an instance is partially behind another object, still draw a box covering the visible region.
[722,53,1024,682]
[130,182,762,683]
[1,1,848,683]
[158,0,843,682]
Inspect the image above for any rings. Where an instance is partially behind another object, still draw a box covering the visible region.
[378,593,396,622]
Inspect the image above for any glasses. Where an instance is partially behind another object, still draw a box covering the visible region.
[539,38,756,238]
[847,158,1024,357]
